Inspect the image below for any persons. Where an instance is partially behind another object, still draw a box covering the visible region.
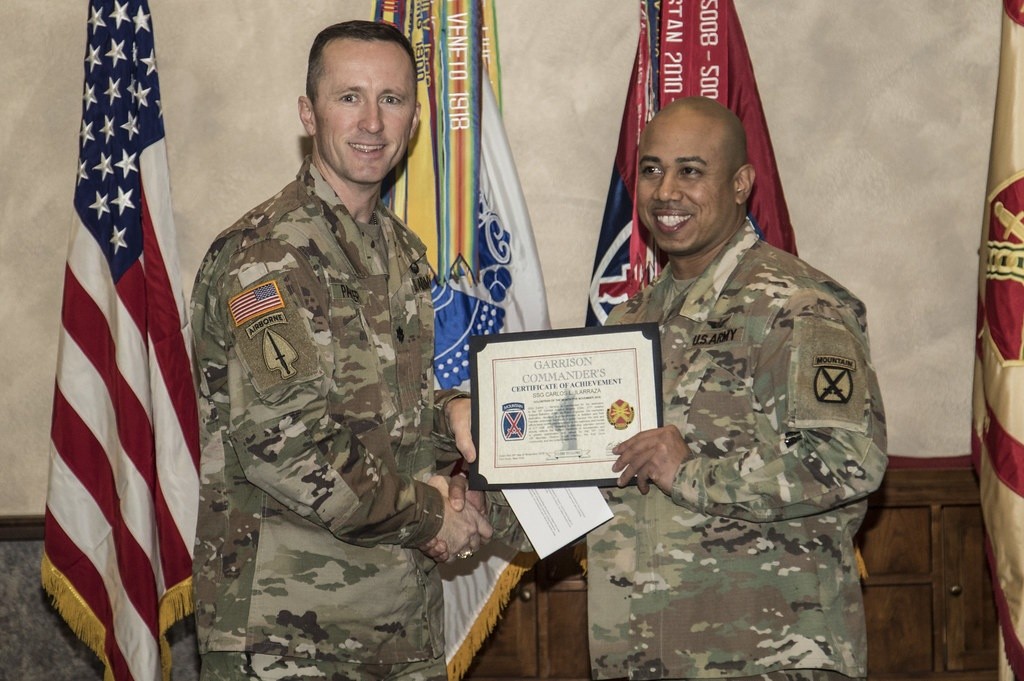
[418,95,888,681]
[190,20,490,681]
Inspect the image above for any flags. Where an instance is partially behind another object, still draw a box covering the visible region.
[584,0,798,330]
[971,0,1024,681]
[371,0,551,681]
[43,1,205,681]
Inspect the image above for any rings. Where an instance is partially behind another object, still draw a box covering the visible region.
[455,547,474,560]
